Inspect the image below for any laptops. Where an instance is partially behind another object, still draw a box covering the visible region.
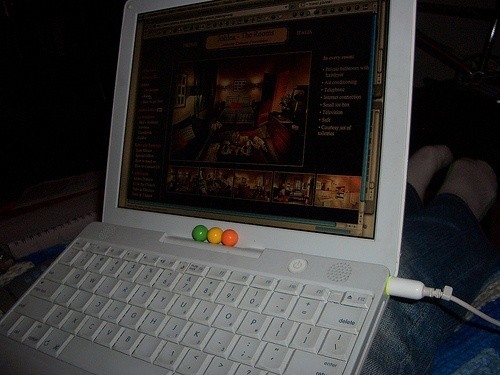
[0,0,418,374]
